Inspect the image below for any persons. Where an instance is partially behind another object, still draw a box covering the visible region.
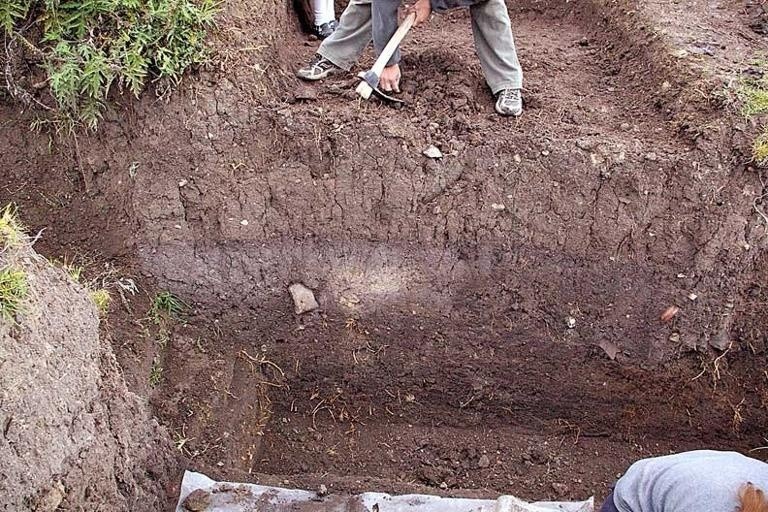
[598,448,767,512]
[310,0,340,38]
[294,0,525,117]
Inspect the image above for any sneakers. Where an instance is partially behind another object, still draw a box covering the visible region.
[302,0,338,37]
[296,54,344,80]
[494,89,522,117]
